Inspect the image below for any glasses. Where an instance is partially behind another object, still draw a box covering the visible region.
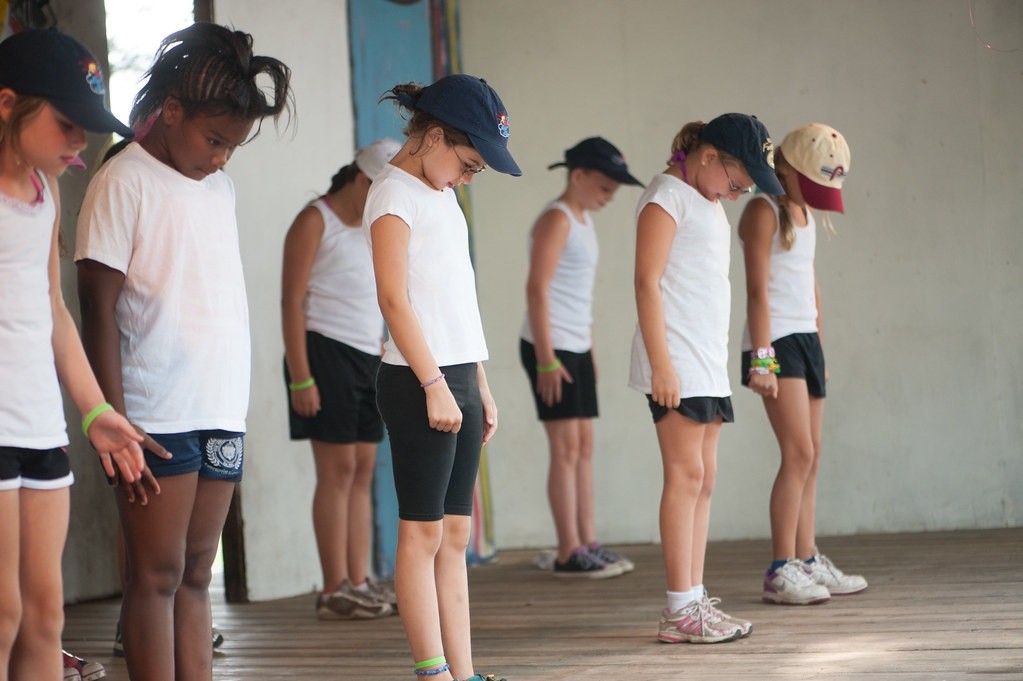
[441,130,488,175]
[717,152,752,195]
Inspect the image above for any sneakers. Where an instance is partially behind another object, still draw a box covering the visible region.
[761,559,831,605]
[314,576,399,621]
[61,647,107,681]
[802,553,868,594]
[551,541,635,579]
[657,594,752,644]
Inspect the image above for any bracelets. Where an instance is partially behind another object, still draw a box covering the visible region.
[749,347,781,373]
[536,359,561,372]
[289,378,315,391]
[81,403,112,438]
[420,374,445,387]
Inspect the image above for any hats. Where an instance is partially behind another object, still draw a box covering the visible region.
[356,138,403,183]
[779,122,850,214]
[699,112,786,195]
[398,74,523,178]
[0,24,136,138]
[547,137,647,189]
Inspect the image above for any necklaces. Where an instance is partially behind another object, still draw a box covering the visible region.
[30,176,42,202]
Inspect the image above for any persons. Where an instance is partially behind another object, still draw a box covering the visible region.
[519,137,646,578]
[628,112,785,643]
[0,28,135,681]
[363,74,522,680]
[74,25,288,681]
[281,138,400,620]
[737,124,867,606]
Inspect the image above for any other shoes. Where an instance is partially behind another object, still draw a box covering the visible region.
[112,623,224,657]
